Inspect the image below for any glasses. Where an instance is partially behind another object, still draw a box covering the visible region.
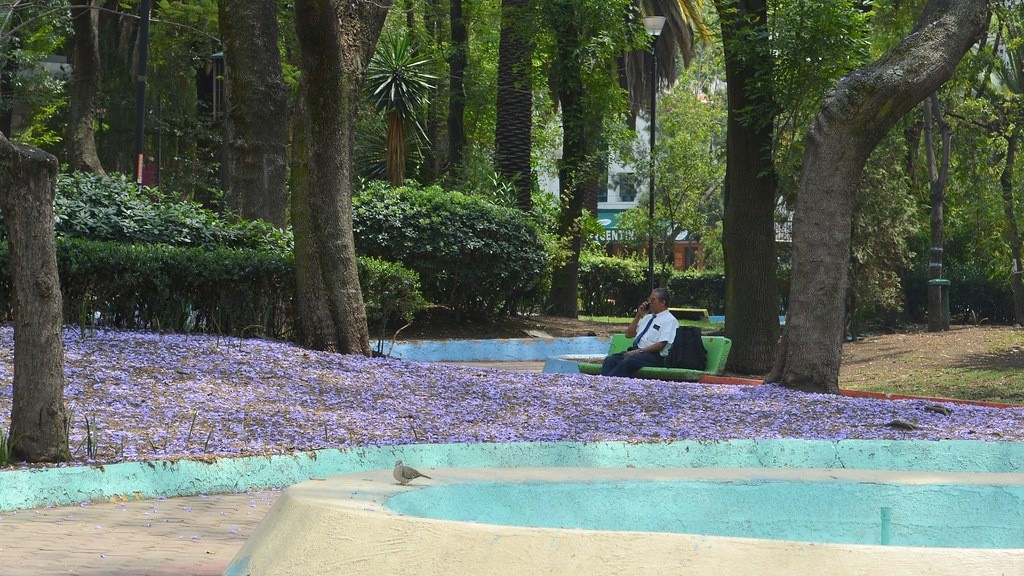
[648,298,661,302]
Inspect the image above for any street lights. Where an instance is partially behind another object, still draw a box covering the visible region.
[643,15,665,313]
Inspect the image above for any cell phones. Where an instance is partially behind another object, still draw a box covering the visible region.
[644,304,650,311]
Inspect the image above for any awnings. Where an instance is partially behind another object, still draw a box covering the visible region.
[597,212,689,244]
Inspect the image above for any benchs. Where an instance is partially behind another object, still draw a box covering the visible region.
[577,333,732,380]
[669,308,710,321]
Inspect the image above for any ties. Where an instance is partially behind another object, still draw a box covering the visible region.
[633,314,656,349]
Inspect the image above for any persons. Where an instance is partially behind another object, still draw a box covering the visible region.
[639,349,643,353]
[601,287,679,377]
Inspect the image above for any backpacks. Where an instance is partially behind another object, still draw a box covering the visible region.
[663,325,709,371]
